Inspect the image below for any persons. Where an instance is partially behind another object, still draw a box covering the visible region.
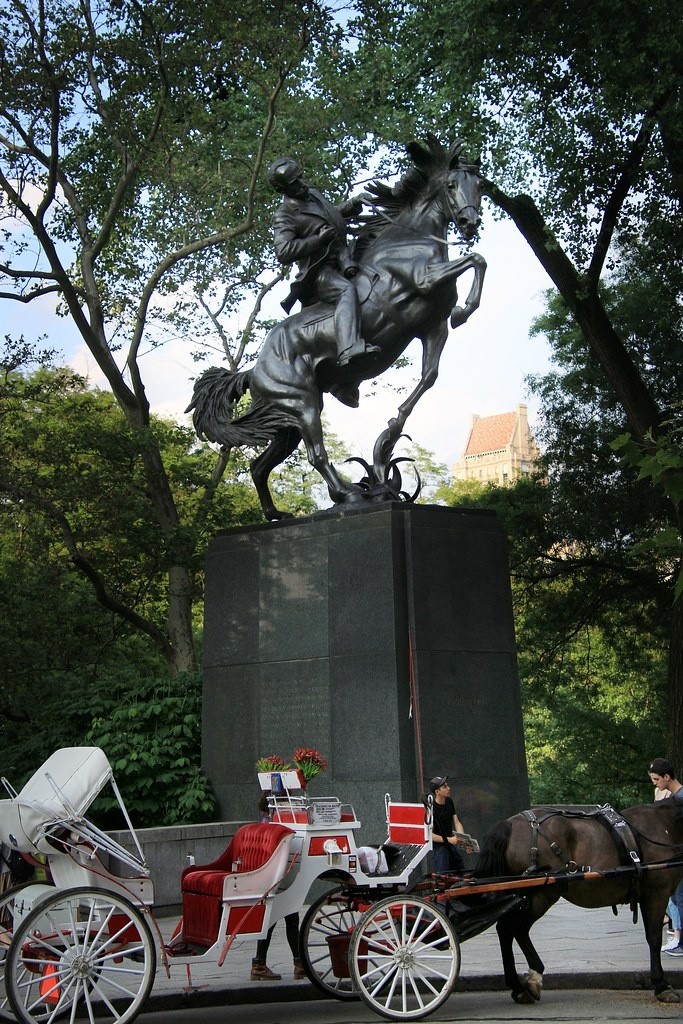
[648,756,683,957]
[268,158,381,371]
[428,775,465,872]
[249,793,325,981]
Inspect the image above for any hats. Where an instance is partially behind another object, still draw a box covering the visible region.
[430,775,450,793]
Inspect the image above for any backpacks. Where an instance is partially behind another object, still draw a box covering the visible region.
[0,845,35,885]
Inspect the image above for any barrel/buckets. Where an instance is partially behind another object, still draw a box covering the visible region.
[325,909,372,978]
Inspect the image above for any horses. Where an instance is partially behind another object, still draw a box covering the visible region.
[467,794,683,1005]
[183,131,488,522]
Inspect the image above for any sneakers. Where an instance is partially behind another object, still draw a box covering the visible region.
[665,945,683,956]
[661,937,679,952]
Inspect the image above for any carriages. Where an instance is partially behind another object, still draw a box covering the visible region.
[3,741,683,1023]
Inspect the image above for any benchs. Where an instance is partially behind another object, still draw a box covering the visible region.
[267,797,360,829]
[47,841,155,910]
[181,824,296,901]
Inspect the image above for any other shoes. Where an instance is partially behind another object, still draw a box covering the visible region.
[667,929,674,934]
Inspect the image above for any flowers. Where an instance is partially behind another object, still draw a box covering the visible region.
[293,748,328,782]
[255,755,290,772]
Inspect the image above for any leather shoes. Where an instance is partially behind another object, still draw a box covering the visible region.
[293,962,324,980]
[251,965,281,981]
